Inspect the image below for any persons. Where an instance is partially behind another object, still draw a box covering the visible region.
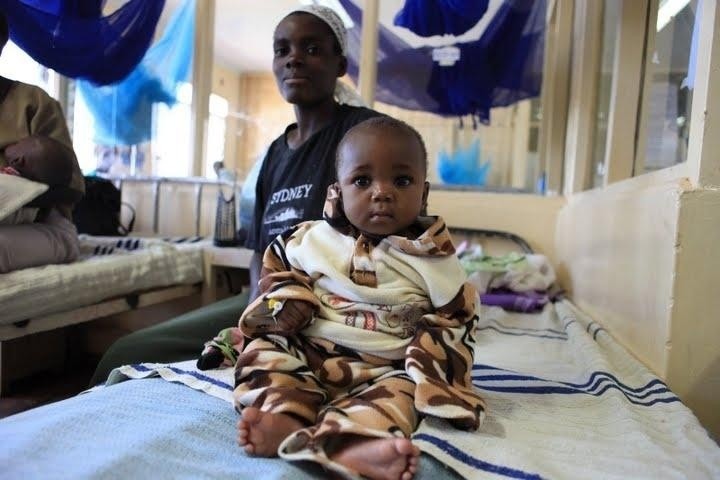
[0,12,86,274]
[86,3,389,389]
[0,135,73,181]
[233,117,489,480]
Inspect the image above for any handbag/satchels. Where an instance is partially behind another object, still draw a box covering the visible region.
[82,175,135,237]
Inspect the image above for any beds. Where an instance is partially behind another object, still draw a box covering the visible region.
[2,228,719,479]
[1,172,232,344]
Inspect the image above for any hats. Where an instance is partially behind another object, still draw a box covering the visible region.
[297,4,347,56]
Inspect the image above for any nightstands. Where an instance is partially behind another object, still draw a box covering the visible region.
[199,239,254,306]
